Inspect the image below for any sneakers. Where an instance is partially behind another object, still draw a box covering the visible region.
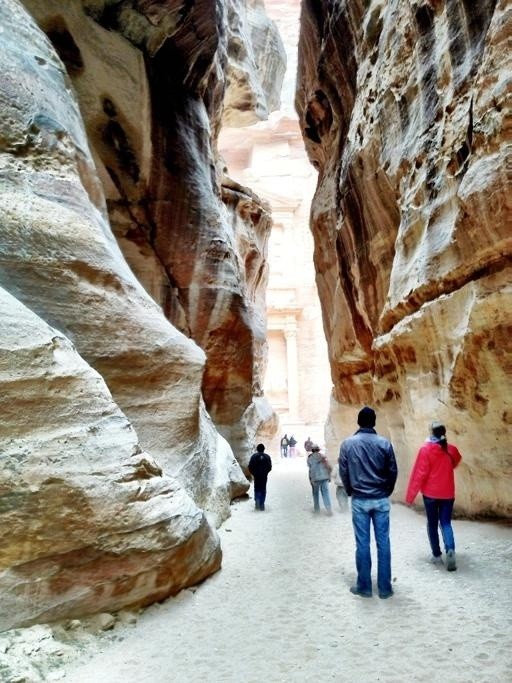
[446,550,457,571]
[431,556,444,565]
[379,591,393,600]
[350,586,372,598]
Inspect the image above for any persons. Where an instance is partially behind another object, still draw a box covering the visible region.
[281,434,289,457]
[331,456,349,512]
[339,406,399,600]
[247,443,272,511]
[289,436,298,457]
[307,444,333,516]
[405,420,462,571]
[304,437,313,455]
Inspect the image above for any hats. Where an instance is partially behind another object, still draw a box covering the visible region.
[312,445,319,449]
[257,444,264,451]
[357,406,376,428]
